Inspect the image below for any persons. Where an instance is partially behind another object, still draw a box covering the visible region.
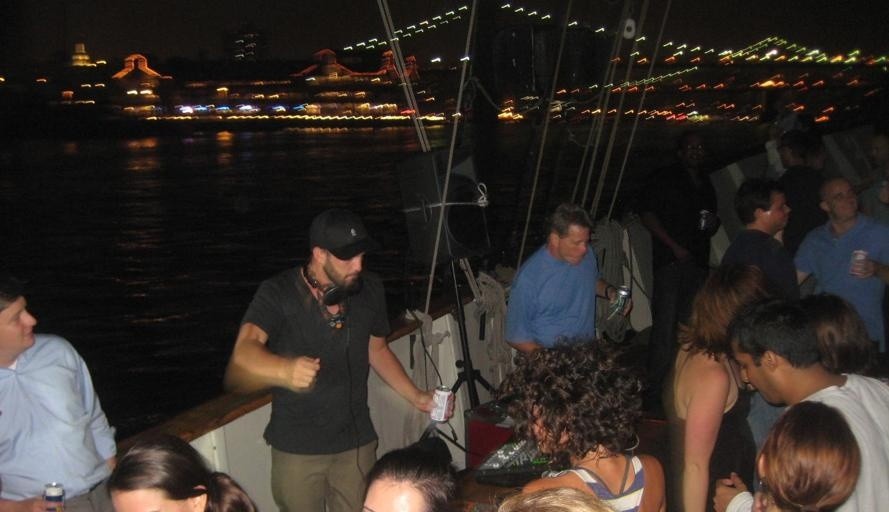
[1,277,118,512]
[355,436,469,512]
[492,94,889,511]
[108,428,256,511]
[229,211,470,512]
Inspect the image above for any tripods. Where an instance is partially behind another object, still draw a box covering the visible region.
[418,261,498,454]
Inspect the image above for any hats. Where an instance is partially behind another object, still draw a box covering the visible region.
[308,209,378,261]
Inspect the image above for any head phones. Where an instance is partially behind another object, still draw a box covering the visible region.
[302,263,343,305]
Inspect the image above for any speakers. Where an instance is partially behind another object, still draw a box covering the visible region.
[396,148,495,269]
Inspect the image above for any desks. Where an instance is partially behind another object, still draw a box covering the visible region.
[448,468,522,512]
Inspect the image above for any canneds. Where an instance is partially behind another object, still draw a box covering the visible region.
[42,481,65,512]
[849,249,869,277]
[696,207,713,232]
[608,284,632,316]
[430,386,452,424]
